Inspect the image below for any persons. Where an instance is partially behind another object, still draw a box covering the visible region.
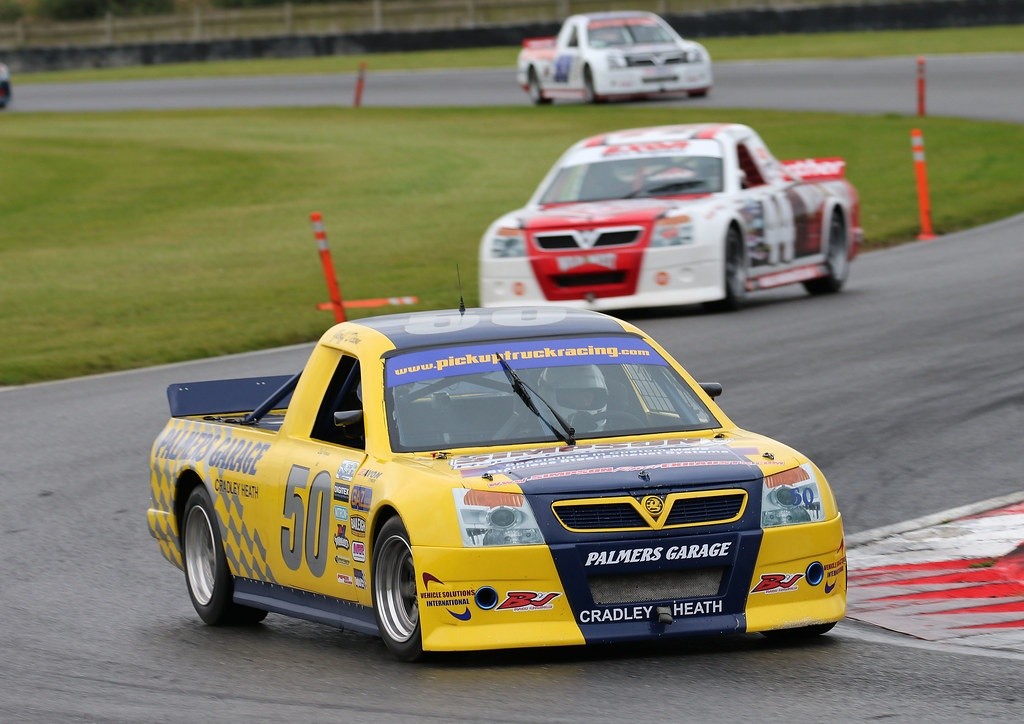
[516,363,610,440]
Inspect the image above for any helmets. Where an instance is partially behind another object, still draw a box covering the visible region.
[534,365,610,436]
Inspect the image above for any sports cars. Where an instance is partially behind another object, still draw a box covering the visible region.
[477,121,865,312]
[516,10,716,107]
[147,305,848,663]
